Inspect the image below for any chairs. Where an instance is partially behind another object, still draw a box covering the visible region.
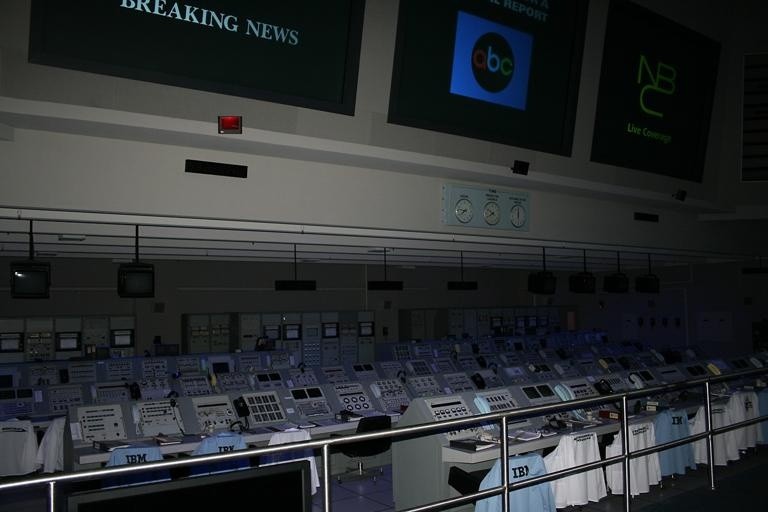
[330,415,392,486]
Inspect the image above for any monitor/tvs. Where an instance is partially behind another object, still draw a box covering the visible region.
[635,273,660,294]
[284,323,302,341]
[55,331,82,351]
[568,273,596,293]
[527,273,557,296]
[263,324,281,340]
[321,322,340,339]
[116,263,156,299]
[10,261,52,300]
[112,329,135,349]
[0,332,25,353]
[602,273,629,294]
[358,321,375,337]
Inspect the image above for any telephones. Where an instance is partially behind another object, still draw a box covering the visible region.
[209,373,218,387]
[90,385,97,400]
[233,396,250,417]
[553,363,565,375]
[656,352,665,361]
[749,356,763,368]
[707,363,721,376]
[130,405,140,424]
[265,354,271,368]
[499,353,508,364]
[590,345,599,354]
[477,356,487,368]
[554,384,571,402]
[473,395,491,414]
[599,359,608,369]
[630,374,644,389]
[130,382,141,399]
[406,361,414,372]
[538,350,546,358]
[370,384,382,399]
[471,373,485,389]
[454,344,460,353]
[599,379,614,393]
[685,349,696,359]
[247,375,255,387]
[472,343,479,354]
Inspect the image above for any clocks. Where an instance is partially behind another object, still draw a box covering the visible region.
[455,199,475,225]
[510,206,526,228]
[482,201,501,225]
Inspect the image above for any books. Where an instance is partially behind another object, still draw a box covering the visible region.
[376,410,401,416]
[507,428,557,441]
[93,441,131,452]
[273,421,315,432]
[599,410,635,420]
[640,401,675,411]
[336,409,364,422]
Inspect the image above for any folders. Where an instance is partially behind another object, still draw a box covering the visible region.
[92,440,132,451]
[564,421,597,428]
